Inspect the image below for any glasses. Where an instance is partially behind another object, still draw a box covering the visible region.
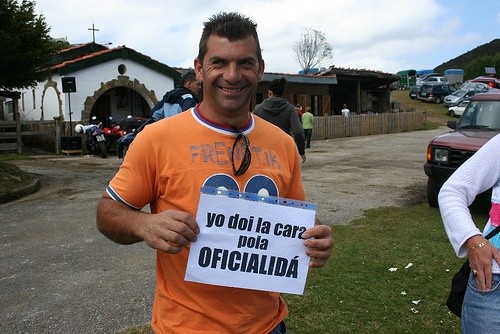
[232,127,252,177]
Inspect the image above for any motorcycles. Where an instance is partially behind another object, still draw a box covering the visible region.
[83,115,136,159]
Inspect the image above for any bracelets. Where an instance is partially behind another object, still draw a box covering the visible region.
[466,239,489,252]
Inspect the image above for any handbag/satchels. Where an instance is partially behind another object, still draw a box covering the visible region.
[445,258,472,318]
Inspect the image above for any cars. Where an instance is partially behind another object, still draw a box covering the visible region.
[409,72,459,104]
[444,75,500,117]
[116,119,156,159]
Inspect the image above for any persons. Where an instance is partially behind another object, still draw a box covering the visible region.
[150,72,201,122]
[301,106,314,148]
[96,12,334,334]
[252,78,306,164]
[391,101,401,112]
[341,104,350,117]
[295,104,303,122]
[489,80,495,88]
[438,133,500,334]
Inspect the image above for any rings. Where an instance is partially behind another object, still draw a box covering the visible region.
[472,269,478,276]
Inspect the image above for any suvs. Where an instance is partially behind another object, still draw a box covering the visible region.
[423,93,500,208]
